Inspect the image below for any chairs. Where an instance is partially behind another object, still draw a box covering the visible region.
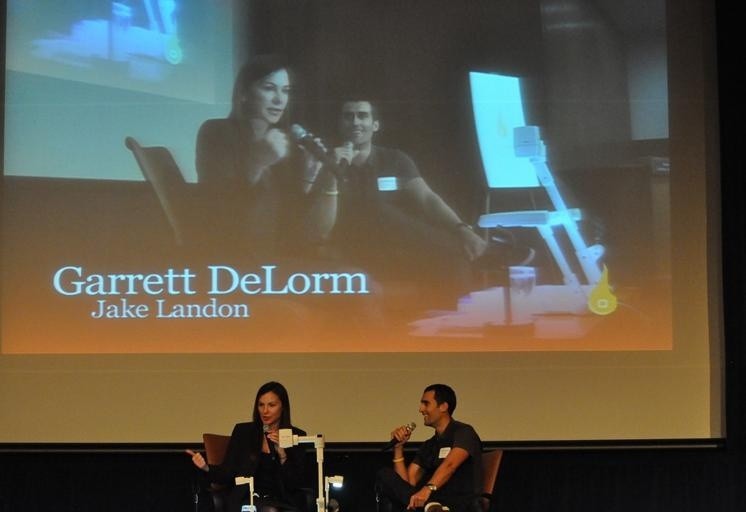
[203,432,315,512]
[124,136,191,247]
[482,450,503,512]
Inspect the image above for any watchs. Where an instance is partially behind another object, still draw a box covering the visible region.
[425,483,438,493]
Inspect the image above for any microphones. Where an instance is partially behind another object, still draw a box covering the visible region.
[379,421,416,455]
[340,140,353,181]
[262,424,279,465]
[289,123,339,176]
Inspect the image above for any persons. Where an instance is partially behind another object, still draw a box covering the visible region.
[376,382,485,511]
[310,90,536,314]
[193,51,392,351]
[184,380,309,512]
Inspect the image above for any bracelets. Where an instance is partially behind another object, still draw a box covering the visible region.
[321,189,338,196]
[455,221,473,230]
[392,456,404,463]
[279,455,288,460]
[296,175,316,184]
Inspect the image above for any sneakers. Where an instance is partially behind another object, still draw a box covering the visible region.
[424,500,450,512]
[472,245,536,281]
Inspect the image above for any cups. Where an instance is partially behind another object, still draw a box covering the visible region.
[507,265,535,316]
[242,506,256,512]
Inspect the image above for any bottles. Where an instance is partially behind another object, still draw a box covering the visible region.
[109,2,133,63]
